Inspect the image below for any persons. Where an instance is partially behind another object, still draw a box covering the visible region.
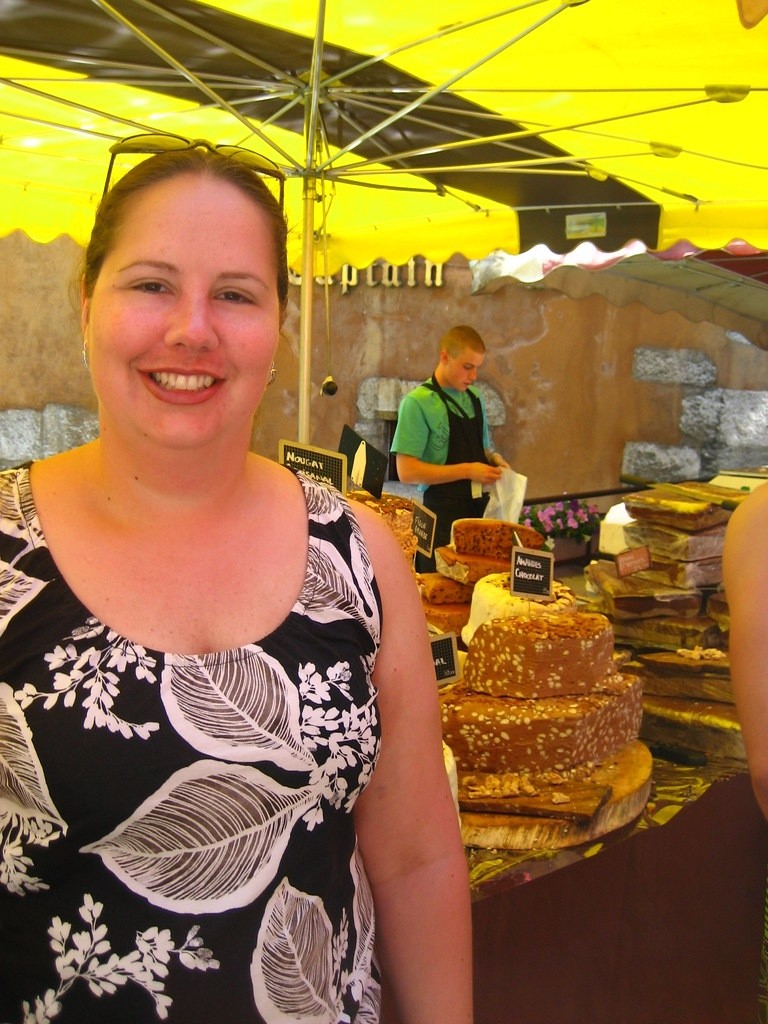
[0,147,476,1024]
[721,480,768,823]
[390,325,512,573]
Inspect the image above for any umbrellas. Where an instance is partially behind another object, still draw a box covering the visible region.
[0,1,768,448]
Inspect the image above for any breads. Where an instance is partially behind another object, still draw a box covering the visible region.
[341,490,643,803]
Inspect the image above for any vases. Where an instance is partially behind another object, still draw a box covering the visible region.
[551,532,588,562]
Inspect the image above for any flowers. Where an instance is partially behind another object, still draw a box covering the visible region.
[517,498,604,553]
